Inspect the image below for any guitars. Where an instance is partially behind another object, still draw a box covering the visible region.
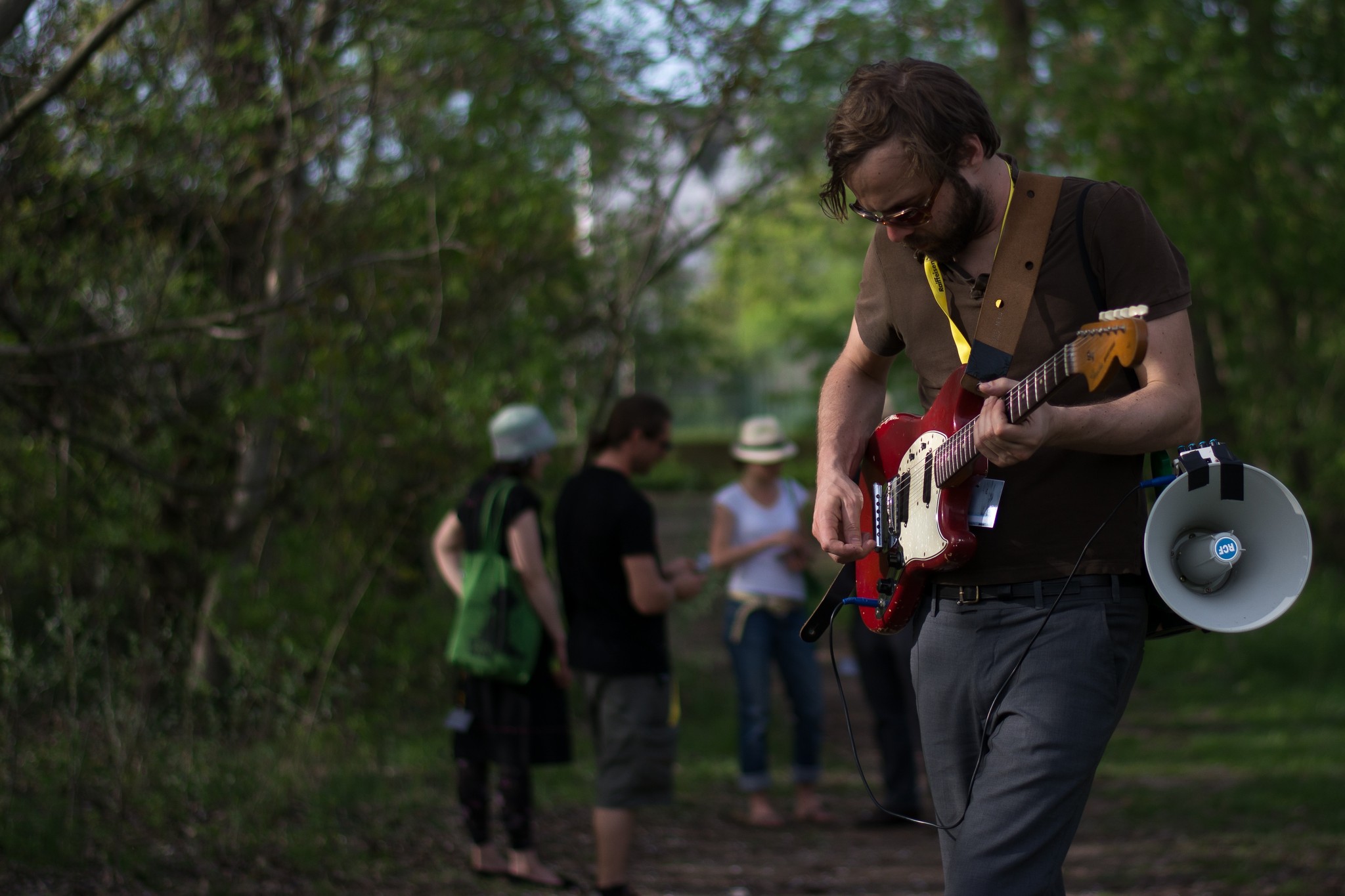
[852,303,1151,638]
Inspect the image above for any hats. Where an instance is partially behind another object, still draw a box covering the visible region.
[731,415,797,466]
[485,402,574,460]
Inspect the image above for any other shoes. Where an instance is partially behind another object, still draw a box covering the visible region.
[597,881,636,896]
[858,804,925,827]
[473,866,509,878]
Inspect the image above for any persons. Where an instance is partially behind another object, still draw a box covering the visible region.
[813,57,1202,896]
[433,399,942,896]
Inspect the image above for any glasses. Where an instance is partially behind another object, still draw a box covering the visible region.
[658,438,672,450]
[848,143,959,229]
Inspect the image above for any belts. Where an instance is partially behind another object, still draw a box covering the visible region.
[936,573,1140,604]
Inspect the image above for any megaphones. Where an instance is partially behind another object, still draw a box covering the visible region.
[1142,461,1313,635]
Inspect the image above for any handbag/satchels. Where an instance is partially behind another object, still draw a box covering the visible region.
[444,477,545,684]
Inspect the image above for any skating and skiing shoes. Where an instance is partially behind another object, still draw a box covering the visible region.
[505,868,578,890]
